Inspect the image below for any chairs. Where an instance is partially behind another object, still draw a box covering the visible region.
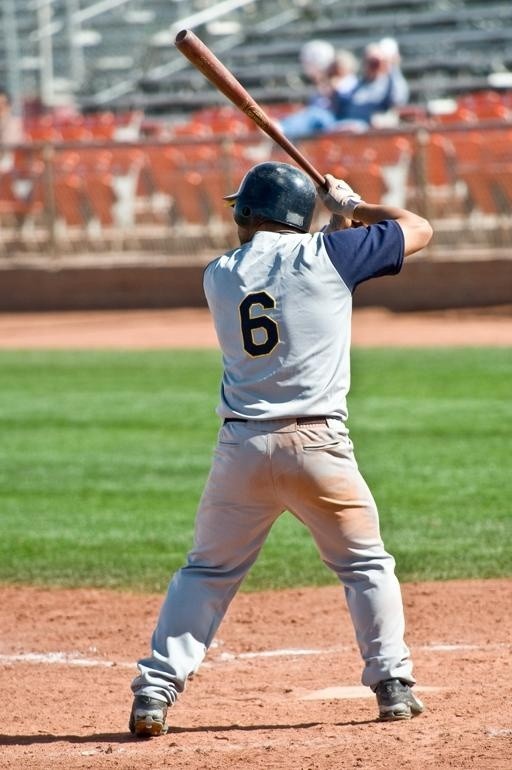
[0,90,512,235]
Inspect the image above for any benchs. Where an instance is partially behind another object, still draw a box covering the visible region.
[111,0,511,100]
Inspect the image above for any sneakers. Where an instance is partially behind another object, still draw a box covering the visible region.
[129,695,169,735]
[376,679,424,721]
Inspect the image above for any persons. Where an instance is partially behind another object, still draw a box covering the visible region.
[296,37,365,123]
[128,161,431,735]
[1,85,38,239]
[277,40,409,140]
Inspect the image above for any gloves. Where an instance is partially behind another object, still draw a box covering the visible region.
[319,212,369,232]
[316,174,365,222]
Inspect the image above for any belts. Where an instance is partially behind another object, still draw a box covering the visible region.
[225,417,327,425]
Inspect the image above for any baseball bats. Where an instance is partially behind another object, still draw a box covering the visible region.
[175,29,362,227]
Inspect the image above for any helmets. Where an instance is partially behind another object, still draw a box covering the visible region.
[223,162,317,231]
